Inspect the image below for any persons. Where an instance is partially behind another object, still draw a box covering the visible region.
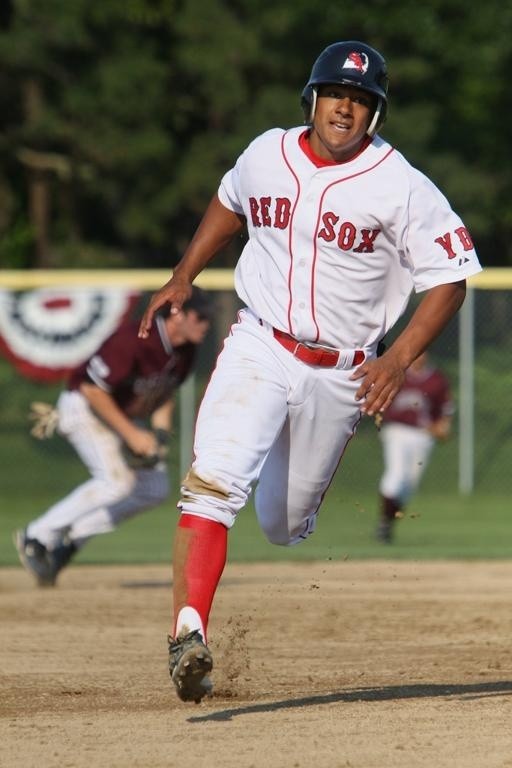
[138,41,483,702]
[373,346,455,544]
[12,286,215,585]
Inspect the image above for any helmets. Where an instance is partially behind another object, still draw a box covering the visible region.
[300,41,388,124]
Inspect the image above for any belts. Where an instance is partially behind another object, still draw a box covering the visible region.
[272,327,365,372]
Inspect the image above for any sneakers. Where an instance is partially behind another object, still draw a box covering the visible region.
[167,633,213,703]
[12,524,78,586]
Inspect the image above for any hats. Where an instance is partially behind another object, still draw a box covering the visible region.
[166,285,223,316]
